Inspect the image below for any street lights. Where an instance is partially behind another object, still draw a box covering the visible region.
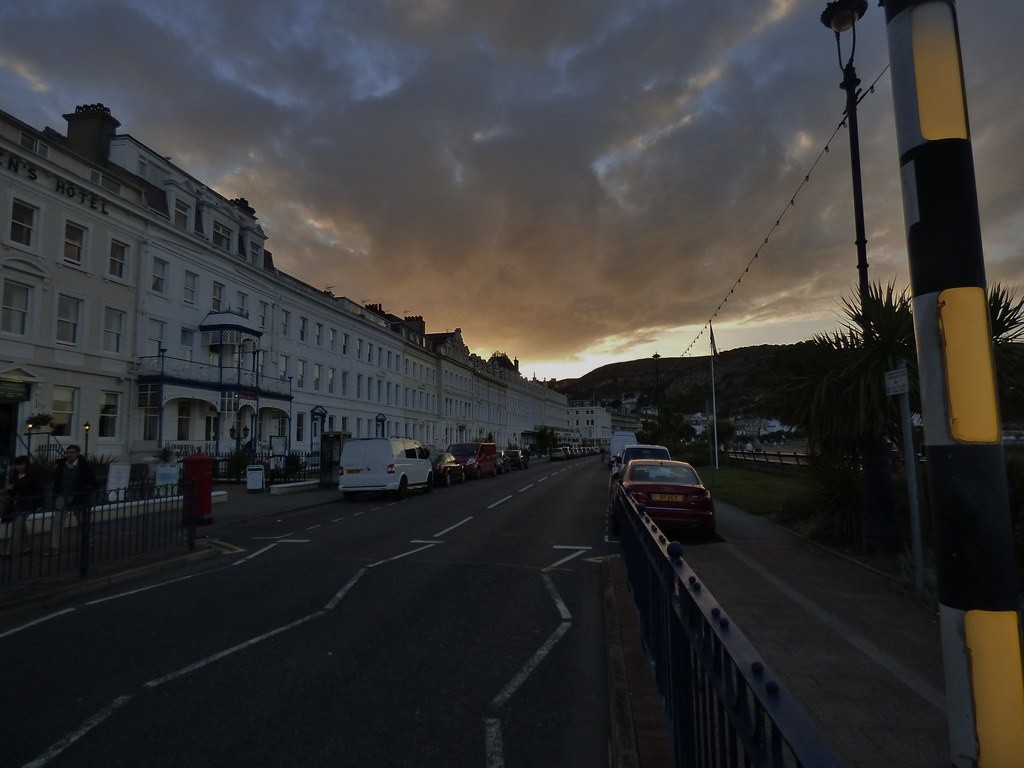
[653,354,661,442]
[820,0,907,554]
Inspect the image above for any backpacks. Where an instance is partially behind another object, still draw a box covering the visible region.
[0,492,16,524]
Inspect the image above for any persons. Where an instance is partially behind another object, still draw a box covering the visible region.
[745,440,768,458]
[0,456,40,559]
[40,444,98,557]
[599,446,606,462]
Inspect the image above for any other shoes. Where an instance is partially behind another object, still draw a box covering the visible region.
[0,554,11,559]
[19,549,32,555]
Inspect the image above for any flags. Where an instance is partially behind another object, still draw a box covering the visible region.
[711,328,719,361]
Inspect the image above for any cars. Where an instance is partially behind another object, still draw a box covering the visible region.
[611,458,716,543]
[430,451,466,486]
[611,445,672,475]
[506,450,529,470]
[548,447,610,462]
[495,450,512,474]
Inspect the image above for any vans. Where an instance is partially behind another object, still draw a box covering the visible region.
[447,443,497,478]
[607,430,641,470]
[338,435,434,500]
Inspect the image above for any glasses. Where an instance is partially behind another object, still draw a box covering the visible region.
[66,451,75,454]
